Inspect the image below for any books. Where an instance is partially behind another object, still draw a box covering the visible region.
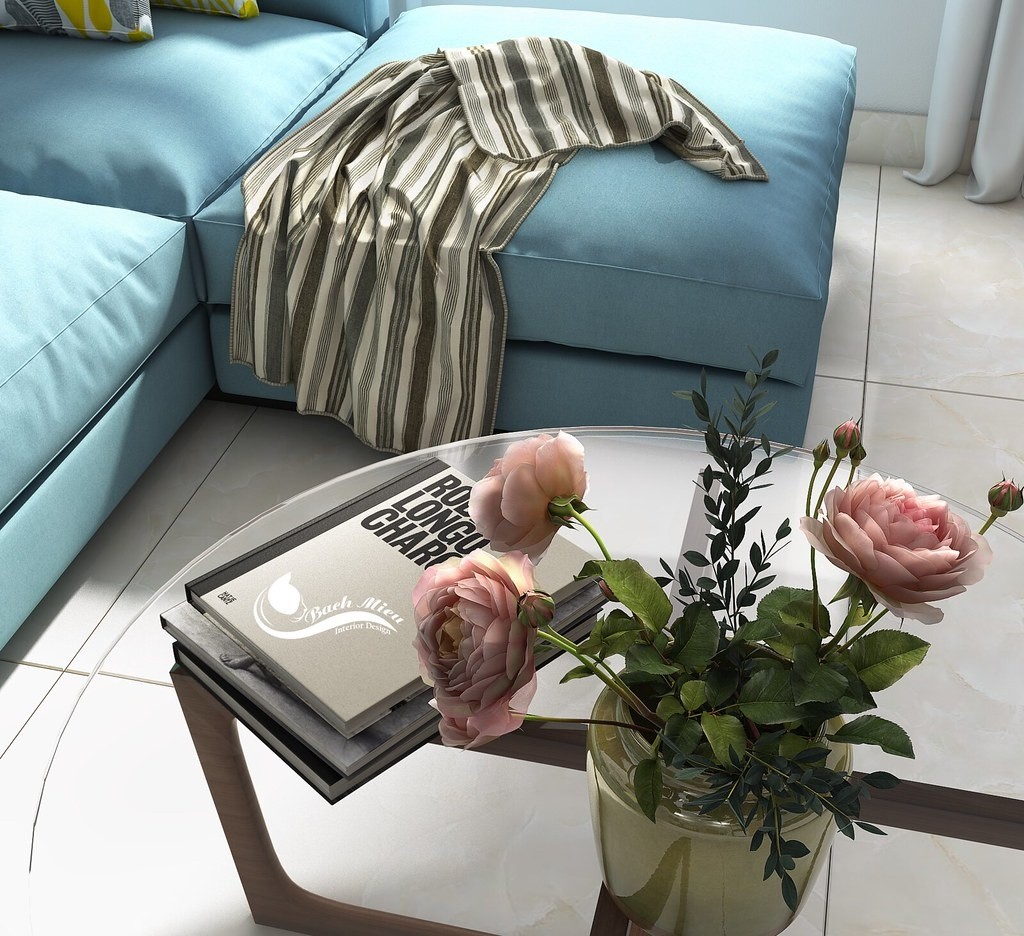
[160,458,621,806]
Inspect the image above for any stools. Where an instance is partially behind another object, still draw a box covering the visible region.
[192,5,859,451]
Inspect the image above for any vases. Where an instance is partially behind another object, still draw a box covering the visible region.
[586,638,855,936]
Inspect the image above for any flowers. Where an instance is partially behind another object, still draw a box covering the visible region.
[412,349,1023,912]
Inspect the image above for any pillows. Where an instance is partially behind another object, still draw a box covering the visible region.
[150,0,259,19]
[0,0,154,42]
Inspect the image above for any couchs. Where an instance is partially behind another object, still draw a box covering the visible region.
[0,0,391,656]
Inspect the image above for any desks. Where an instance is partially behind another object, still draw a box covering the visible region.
[35,426,1024,936]
